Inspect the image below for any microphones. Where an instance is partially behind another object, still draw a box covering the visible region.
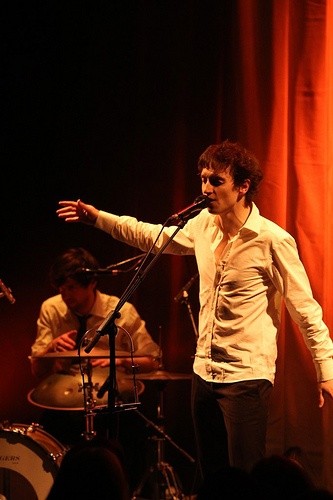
[174,273,199,303]
[72,267,128,281]
[164,195,210,227]
[0,279,16,304]
[97,375,109,399]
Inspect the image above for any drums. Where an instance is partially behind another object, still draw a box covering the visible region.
[0,420,69,500]
[27,363,145,411]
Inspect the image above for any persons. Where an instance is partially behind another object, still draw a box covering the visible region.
[57,142,333,500]
[197,453,333,500]
[30,244,163,500]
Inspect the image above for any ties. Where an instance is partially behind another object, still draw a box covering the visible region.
[75,315,88,364]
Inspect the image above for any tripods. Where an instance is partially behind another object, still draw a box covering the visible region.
[129,382,188,500]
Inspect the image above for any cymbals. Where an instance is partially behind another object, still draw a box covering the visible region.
[120,370,192,381]
[32,347,148,359]
